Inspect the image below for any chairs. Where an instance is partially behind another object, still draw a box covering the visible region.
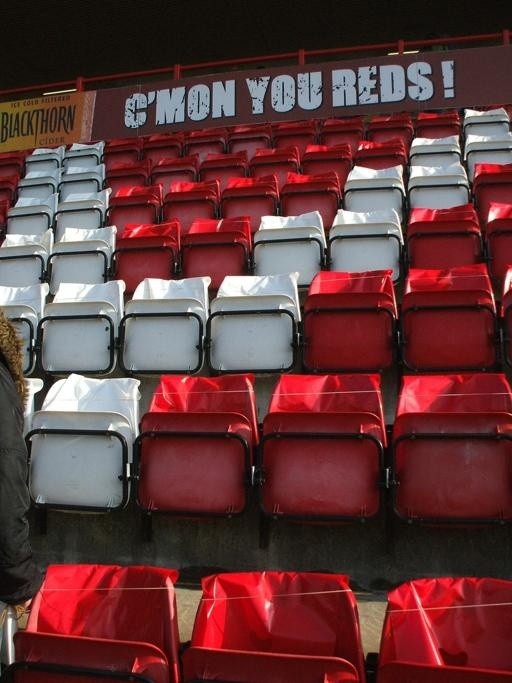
[23,375,141,517]
[389,373,511,521]
[0,564,181,682]
[132,372,261,516]
[0,105,512,371]
[365,576,512,683]
[259,372,388,518]
[182,572,366,683]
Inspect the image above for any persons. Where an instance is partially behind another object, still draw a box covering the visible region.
[0,308,44,615]
